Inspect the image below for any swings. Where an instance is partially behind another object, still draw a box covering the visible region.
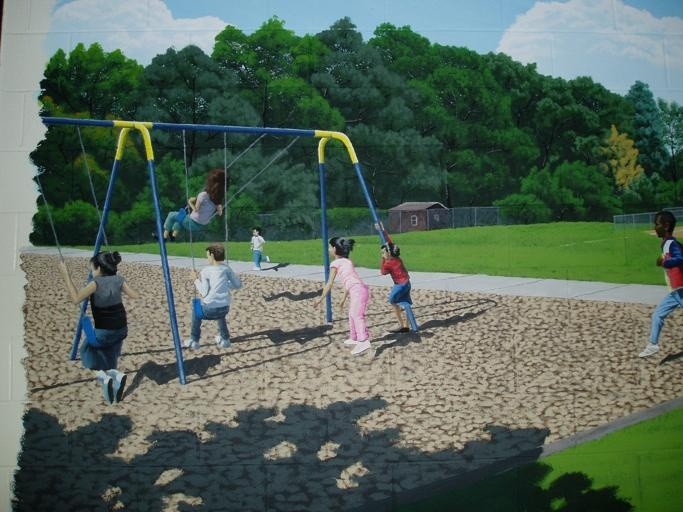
[176,133,301,232]
[182,130,228,320]
[37,124,127,349]
